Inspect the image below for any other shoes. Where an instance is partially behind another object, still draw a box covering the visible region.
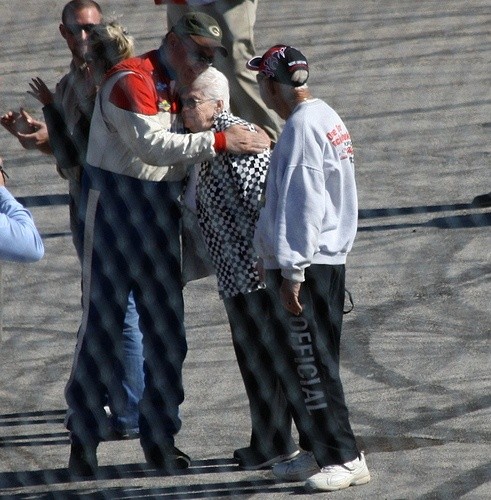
[68,442,99,478]
[233,445,300,470]
[144,446,191,472]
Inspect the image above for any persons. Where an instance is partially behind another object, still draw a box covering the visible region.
[0,156,45,264]
[252,43,372,491]
[0,0,105,271]
[62,12,272,479]
[153,0,284,151]
[25,19,145,442]
[178,65,302,470]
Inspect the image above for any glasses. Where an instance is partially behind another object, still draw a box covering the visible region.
[177,36,216,66]
[256,74,271,83]
[64,23,100,35]
[178,98,216,109]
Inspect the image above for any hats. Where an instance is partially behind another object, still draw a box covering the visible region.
[246,44,309,87]
[171,12,228,58]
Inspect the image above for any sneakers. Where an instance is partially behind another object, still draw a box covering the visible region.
[305,451,370,492]
[272,451,321,481]
